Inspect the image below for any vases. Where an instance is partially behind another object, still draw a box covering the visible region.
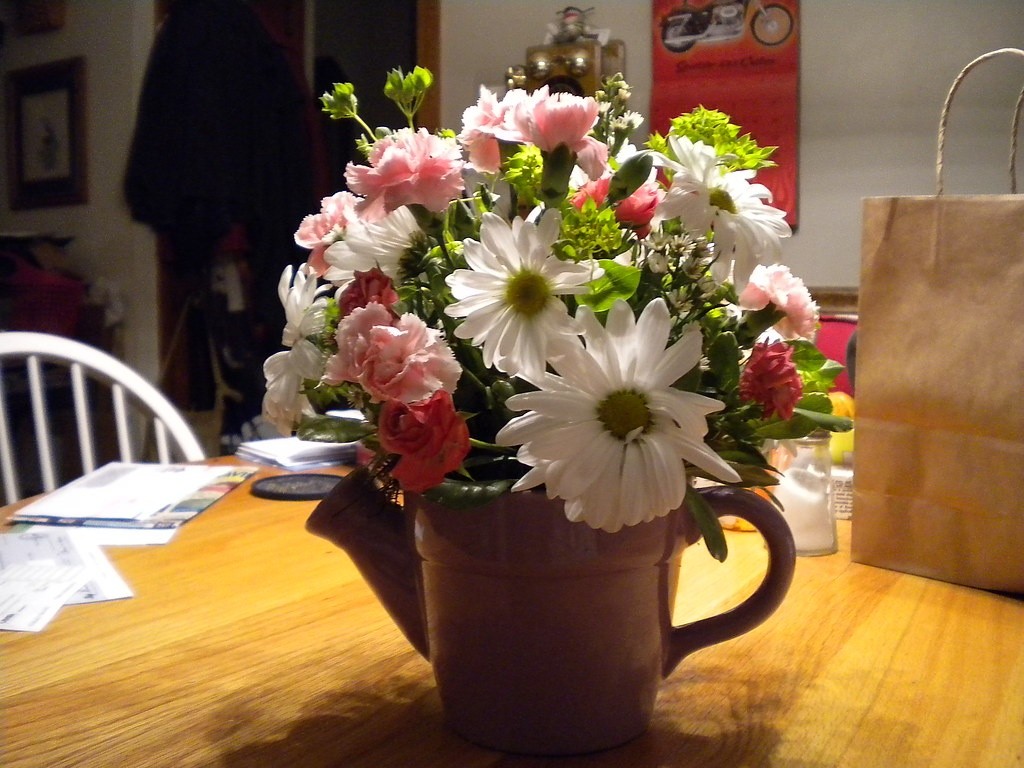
[305,462,797,755]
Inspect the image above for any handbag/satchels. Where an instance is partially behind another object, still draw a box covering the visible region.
[850,48,1024,590]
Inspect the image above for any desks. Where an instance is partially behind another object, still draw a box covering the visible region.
[0,457,1024,768]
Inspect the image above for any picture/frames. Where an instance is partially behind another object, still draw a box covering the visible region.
[7,55,90,210]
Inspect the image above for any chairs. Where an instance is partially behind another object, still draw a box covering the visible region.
[0,331,206,506]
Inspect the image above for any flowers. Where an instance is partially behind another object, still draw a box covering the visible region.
[263,66,853,562]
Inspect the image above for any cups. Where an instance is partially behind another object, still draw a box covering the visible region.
[768,431,838,558]
